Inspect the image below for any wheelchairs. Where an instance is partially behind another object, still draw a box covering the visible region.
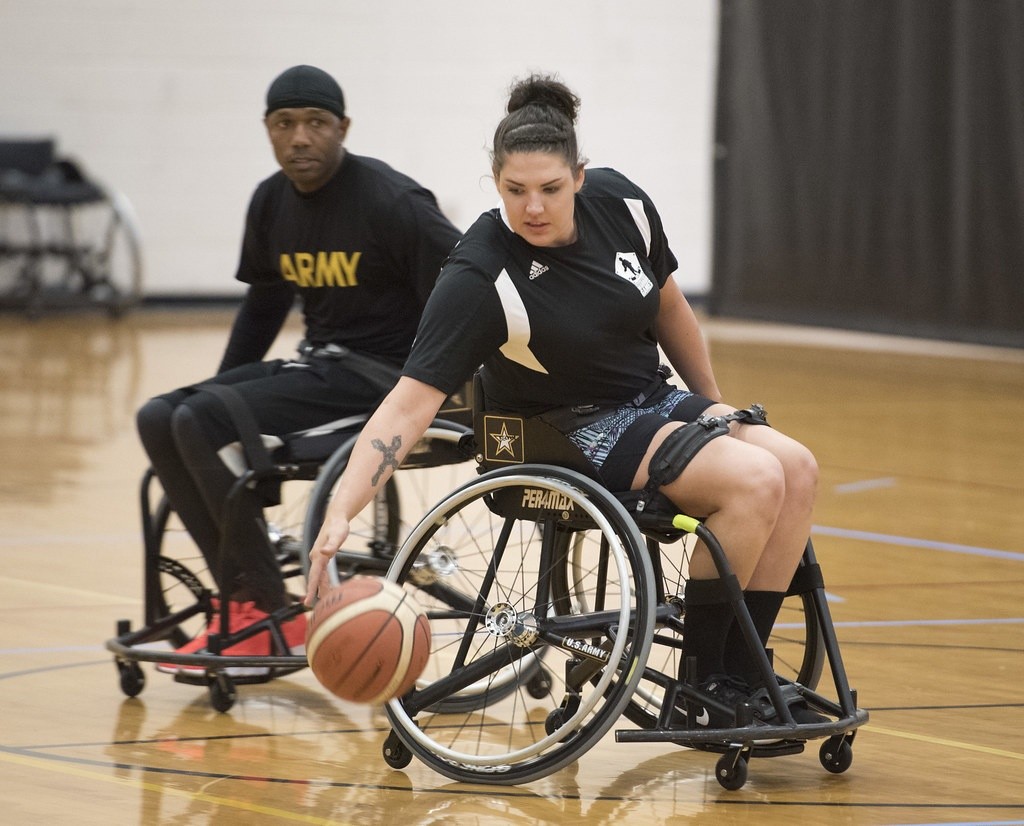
[102,363,872,792]
[0,137,141,314]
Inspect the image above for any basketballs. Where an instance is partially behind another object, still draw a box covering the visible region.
[303,575,432,708]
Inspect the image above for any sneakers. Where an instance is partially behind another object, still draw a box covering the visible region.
[156,589,311,679]
[670,672,832,757]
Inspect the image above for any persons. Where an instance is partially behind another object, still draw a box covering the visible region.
[135,65,509,679]
[303,76,835,742]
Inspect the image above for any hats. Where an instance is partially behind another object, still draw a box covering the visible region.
[265,65,345,120]
